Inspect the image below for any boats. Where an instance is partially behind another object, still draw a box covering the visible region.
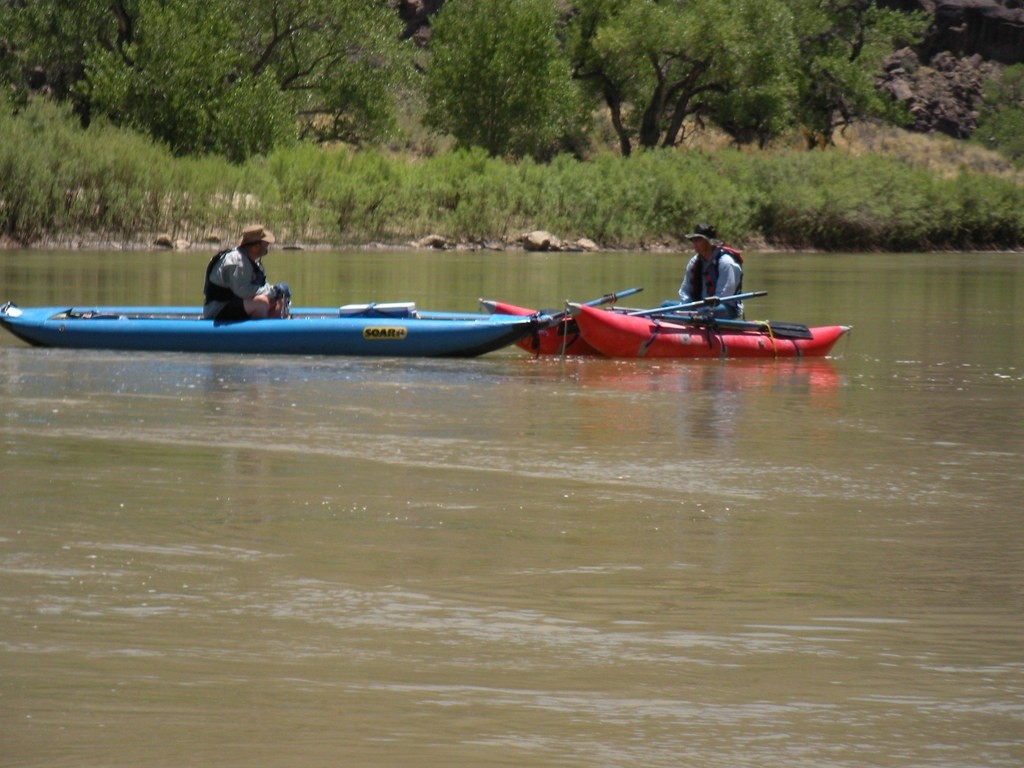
[0,301,547,360]
[480,296,615,359]
[566,298,855,358]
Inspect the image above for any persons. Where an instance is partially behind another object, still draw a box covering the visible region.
[658,223,747,325]
[202,222,288,317]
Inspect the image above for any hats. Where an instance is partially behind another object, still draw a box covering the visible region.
[685,224,717,241]
[236,224,275,249]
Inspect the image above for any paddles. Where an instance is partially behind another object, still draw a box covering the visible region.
[623,289,769,317]
[606,305,813,339]
[528,287,646,332]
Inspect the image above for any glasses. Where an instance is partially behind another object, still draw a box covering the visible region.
[688,236,703,243]
